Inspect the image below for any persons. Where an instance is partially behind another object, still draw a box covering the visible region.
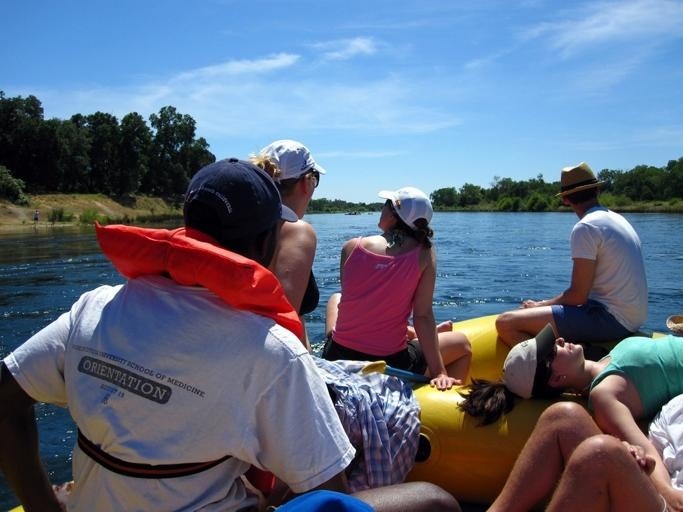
[322,186,471,391]
[496,160,648,359]
[247,141,326,353]
[454,322,683,512]
[34,210,40,226]
[485,392,683,512]
[0,159,460,511]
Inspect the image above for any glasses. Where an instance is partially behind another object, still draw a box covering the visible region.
[301,170,320,187]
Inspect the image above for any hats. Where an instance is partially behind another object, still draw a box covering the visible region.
[185,158,298,248]
[554,161,606,197]
[378,186,433,230]
[500,322,556,402]
[256,139,327,183]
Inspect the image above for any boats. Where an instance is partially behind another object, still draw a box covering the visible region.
[314,301,676,507]
